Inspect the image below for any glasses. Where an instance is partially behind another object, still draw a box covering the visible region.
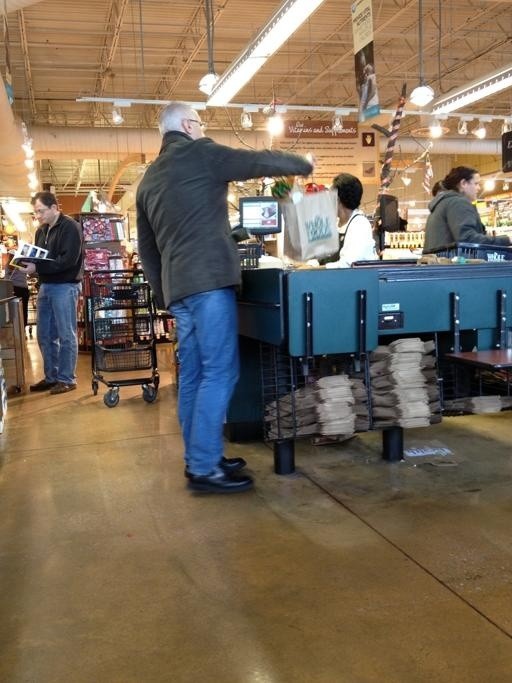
[190,119,207,131]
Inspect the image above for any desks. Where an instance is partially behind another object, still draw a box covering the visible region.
[444,350,512,398]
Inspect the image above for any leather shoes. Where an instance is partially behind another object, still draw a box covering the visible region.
[187,458,253,494]
[30,380,76,395]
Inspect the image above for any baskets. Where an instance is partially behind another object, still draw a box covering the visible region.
[422,241,512,260]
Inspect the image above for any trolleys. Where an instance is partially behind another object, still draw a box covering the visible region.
[89,268,159,407]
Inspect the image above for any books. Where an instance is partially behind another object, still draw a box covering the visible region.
[8,241,57,271]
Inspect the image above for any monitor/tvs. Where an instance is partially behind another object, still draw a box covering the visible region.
[239,197,281,233]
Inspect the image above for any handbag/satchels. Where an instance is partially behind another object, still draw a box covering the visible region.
[280,173,339,262]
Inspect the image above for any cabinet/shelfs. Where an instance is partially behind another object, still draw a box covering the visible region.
[69,212,172,350]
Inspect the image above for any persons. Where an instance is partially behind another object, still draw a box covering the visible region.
[4,258,30,327]
[422,166,512,252]
[372,217,408,261]
[288,171,379,269]
[136,101,316,494]
[356,49,378,111]
[19,191,85,396]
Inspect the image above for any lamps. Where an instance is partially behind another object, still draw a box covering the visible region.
[22,137,38,197]
[410,0,434,106]
[402,174,412,186]
[471,120,487,140]
[113,104,123,125]
[428,119,443,139]
[266,80,284,136]
[207,0,324,106]
[199,0,218,95]
